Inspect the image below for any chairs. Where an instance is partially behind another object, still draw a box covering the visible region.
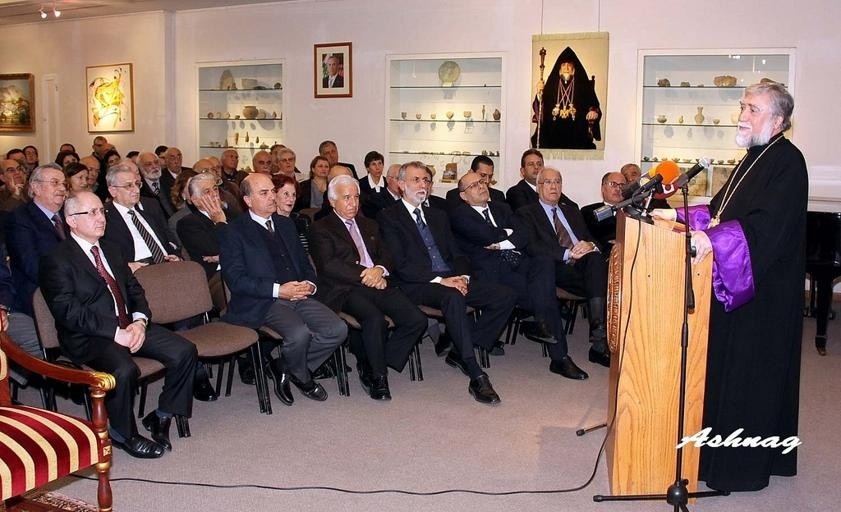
[0,196,589,422]
[0,328,120,512]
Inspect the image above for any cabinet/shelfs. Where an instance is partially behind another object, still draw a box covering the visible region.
[641,85,787,167]
[199,87,283,149]
[389,84,501,158]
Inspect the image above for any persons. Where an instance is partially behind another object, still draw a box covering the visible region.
[533,47,602,149]
[323,56,343,88]
[650,78,808,492]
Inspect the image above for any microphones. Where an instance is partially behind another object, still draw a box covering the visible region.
[593,155,712,223]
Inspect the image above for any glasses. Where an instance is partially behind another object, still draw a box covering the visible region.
[537,179,562,189]
[462,179,485,192]
[111,181,144,192]
[37,178,69,190]
[71,207,107,217]
[603,180,628,191]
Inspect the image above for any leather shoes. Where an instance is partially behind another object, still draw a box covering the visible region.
[191,372,220,403]
[106,428,166,459]
[262,338,392,408]
[143,410,173,453]
[432,315,610,405]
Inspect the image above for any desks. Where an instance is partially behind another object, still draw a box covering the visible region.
[806,211,840,356]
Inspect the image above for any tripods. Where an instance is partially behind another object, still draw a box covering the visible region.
[593,187,731,512]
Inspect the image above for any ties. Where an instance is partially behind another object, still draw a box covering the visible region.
[151,182,159,194]
[51,214,67,241]
[482,209,494,228]
[127,209,168,265]
[413,208,428,231]
[265,220,275,233]
[346,217,368,266]
[551,208,577,267]
[90,245,129,328]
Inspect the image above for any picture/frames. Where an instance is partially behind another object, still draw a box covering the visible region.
[86,62,134,134]
[313,42,353,99]
[0,73,36,133]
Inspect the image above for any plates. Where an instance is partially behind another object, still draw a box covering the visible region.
[438,61,460,82]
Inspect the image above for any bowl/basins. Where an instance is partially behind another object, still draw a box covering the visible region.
[400,110,471,119]
[641,156,741,166]
[713,76,736,87]
[713,119,719,124]
[239,78,257,90]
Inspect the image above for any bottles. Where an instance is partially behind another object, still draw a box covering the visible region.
[222,139,227,147]
[679,115,683,123]
[492,109,499,120]
[243,131,259,143]
[656,114,667,122]
[256,109,277,119]
[214,112,230,119]
[243,105,258,119]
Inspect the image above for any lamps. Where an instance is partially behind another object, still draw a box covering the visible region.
[52,1,61,17]
[36,4,47,19]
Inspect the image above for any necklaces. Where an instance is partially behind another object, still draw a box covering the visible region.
[706,134,783,229]
[551,78,577,120]
